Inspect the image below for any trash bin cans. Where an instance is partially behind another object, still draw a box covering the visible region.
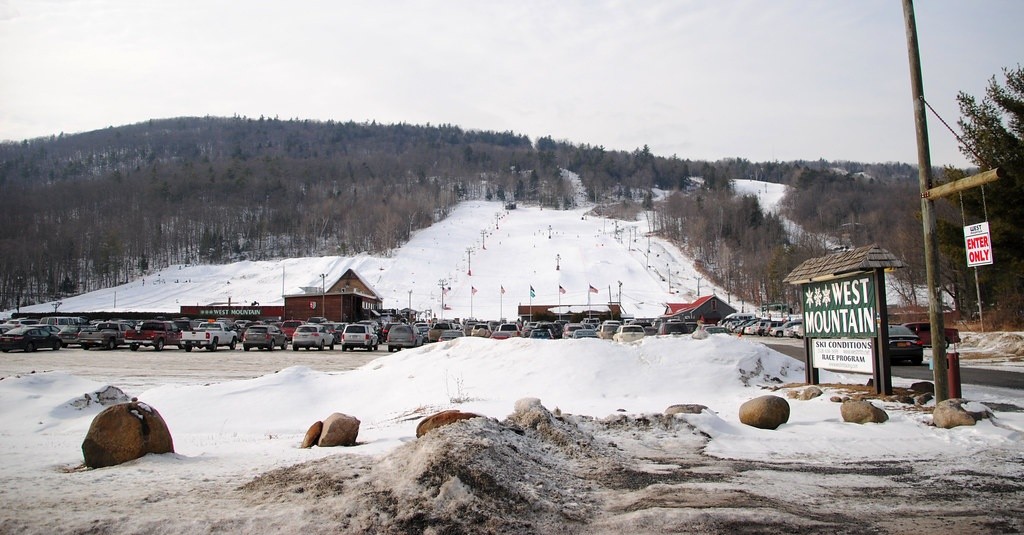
[946,352,961,399]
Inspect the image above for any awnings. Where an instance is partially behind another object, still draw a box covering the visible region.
[371,310,381,317]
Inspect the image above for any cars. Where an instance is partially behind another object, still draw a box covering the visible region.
[0,315,522,341]
[521,322,539,338]
[438,330,466,342]
[241,325,289,351]
[530,328,555,341]
[0,326,63,353]
[900,322,961,348]
[536,312,804,340]
[56,325,95,348]
[292,325,335,351]
[612,324,647,345]
[387,325,424,352]
[887,324,924,365]
[573,329,599,341]
[656,321,690,337]
[340,324,379,352]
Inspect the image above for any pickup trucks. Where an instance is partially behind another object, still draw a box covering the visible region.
[124,320,185,351]
[76,322,136,350]
[178,320,238,352]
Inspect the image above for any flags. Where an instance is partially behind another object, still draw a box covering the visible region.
[590,285,598,293]
[559,285,566,294]
[530,286,535,298]
[472,287,477,294]
[442,287,448,294]
[501,286,505,293]
